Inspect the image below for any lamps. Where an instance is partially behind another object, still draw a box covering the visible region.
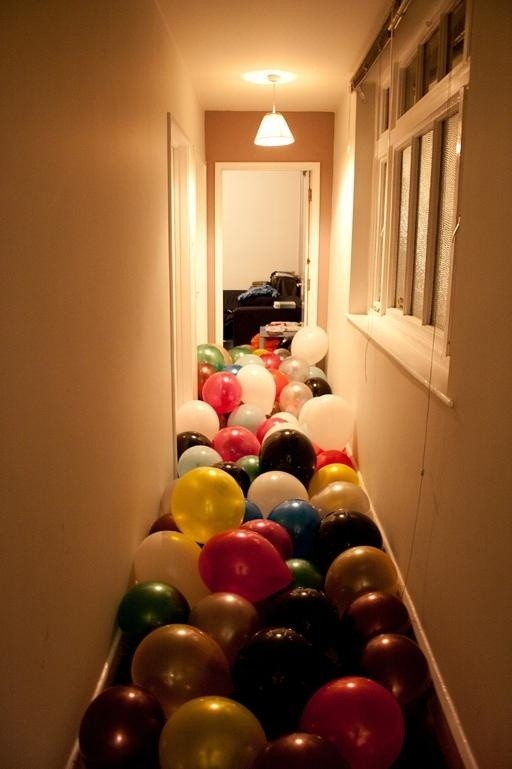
[253,73,296,150]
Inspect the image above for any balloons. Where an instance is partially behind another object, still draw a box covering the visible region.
[291,324,328,365]
[77,345,432,768]
